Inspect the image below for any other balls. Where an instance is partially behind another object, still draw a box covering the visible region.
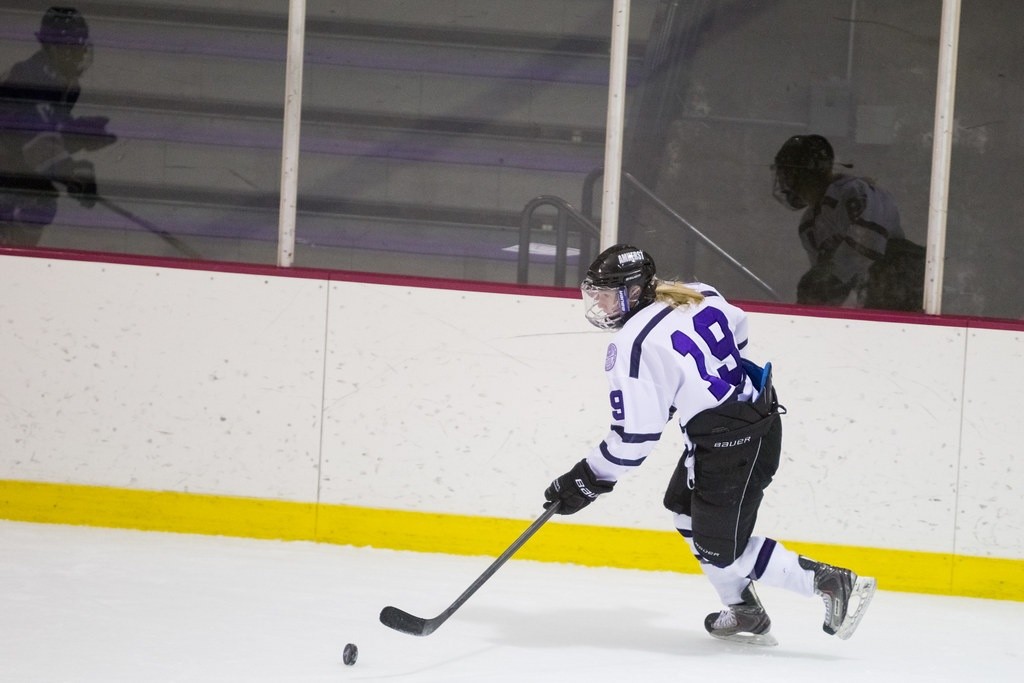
[342,644,359,666]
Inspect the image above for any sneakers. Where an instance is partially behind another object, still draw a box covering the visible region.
[799,554,877,640]
[704,578,778,646]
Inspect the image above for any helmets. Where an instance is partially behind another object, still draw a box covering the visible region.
[581,244,657,328]
[774,135,834,209]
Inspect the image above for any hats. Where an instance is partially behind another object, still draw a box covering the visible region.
[34,7,91,45]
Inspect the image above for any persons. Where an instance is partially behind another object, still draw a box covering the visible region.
[0,5,118,249]
[770,133,906,307]
[544,243,876,646]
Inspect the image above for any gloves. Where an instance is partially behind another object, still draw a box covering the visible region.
[543,458,617,515]
[53,158,98,209]
[70,116,117,153]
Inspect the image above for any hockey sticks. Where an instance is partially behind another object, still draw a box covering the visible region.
[378,499,561,638]
[95,195,203,261]
